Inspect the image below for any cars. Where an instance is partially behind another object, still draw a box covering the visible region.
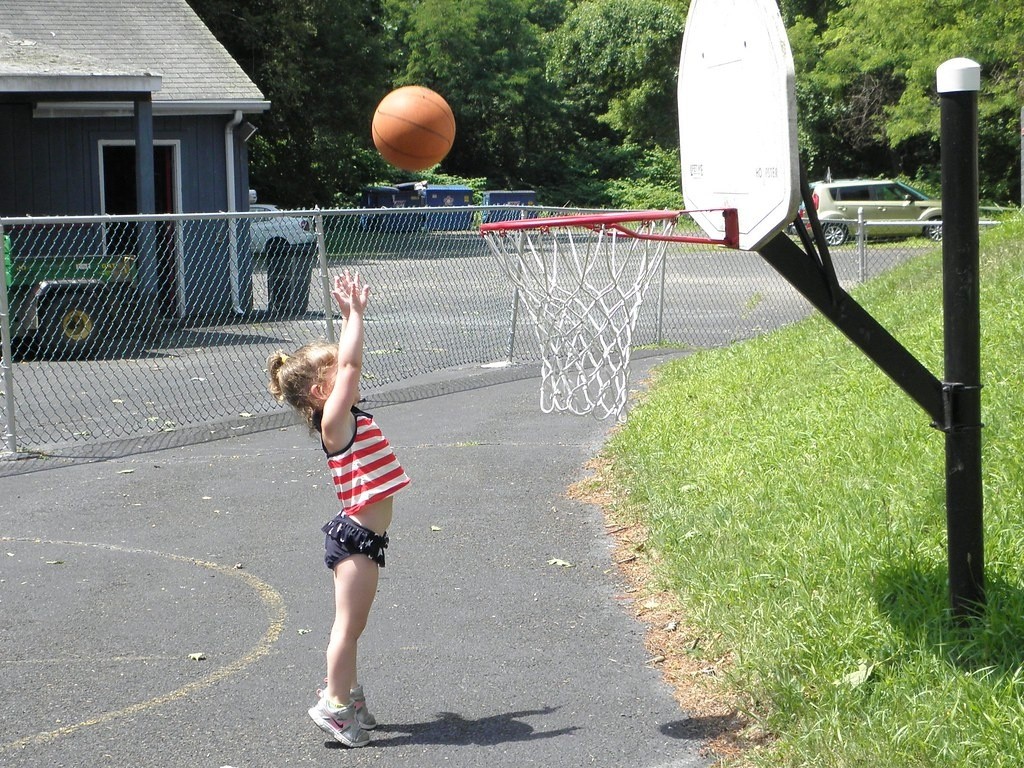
[784,177,943,247]
[249,202,318,256]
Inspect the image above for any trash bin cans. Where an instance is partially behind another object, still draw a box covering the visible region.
[481,189,537,224]
[423,184,475,231]
[361,182,422,235]
[264,230,320,316]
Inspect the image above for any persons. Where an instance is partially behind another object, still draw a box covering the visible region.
[265,270,412,748]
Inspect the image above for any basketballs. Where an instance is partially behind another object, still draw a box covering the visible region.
[372,84,455,171]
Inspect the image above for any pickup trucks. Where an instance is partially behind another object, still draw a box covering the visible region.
[0,235,139,361]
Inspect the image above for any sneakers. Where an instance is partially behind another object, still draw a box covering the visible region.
[317,678,379,728]
[308,689,371,747]
